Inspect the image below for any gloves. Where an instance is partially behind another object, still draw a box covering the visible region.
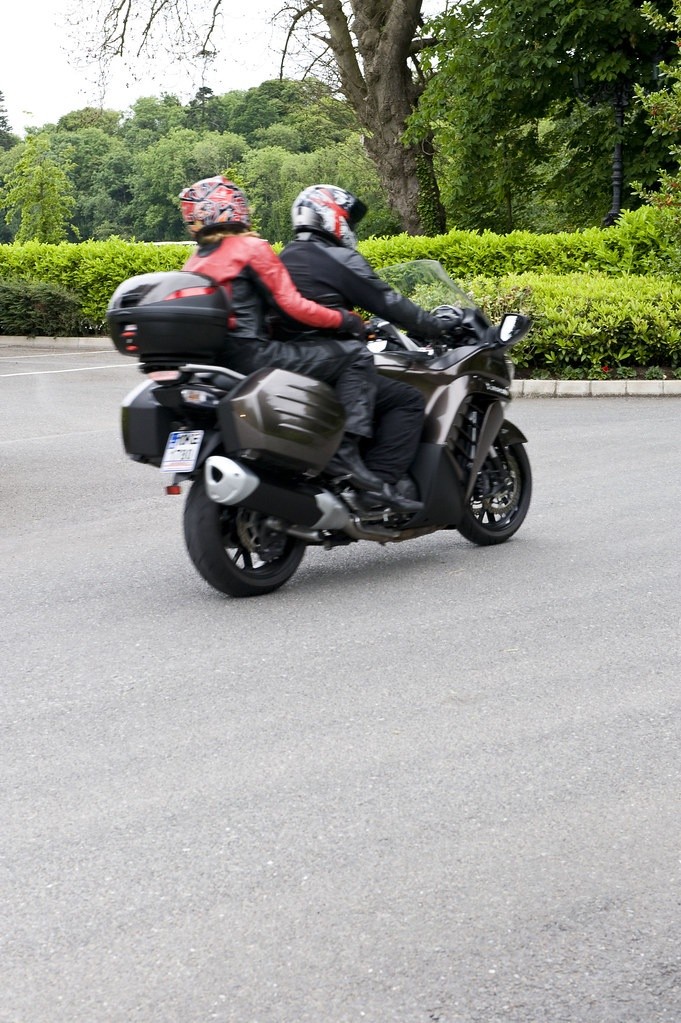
[328,306,366,341]
[428,316,462,344]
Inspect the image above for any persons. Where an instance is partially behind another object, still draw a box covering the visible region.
[265,184,465,511]
[179,176,385,493]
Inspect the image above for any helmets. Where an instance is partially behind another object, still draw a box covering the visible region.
[180,174,251,242]
[291,183,369,250]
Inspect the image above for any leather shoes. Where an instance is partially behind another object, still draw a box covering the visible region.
[324,438,383,491]
[361,481,424,512]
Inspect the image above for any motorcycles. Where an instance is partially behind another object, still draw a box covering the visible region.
[105,259,533,597]
